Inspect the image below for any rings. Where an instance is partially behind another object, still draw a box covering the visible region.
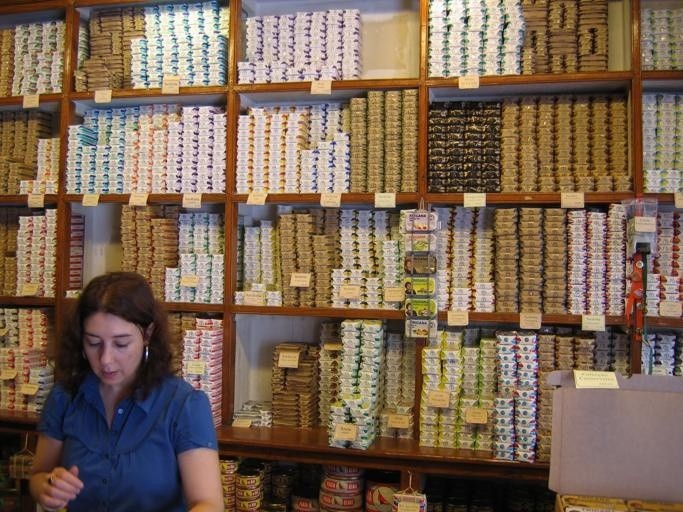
[47,474,54,485]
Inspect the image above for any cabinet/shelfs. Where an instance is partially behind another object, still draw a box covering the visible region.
[0,0,683,512]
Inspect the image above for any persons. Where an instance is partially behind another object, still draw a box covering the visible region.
[29,272,225,512]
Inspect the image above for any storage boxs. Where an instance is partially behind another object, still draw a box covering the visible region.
[545,370,683,501]
